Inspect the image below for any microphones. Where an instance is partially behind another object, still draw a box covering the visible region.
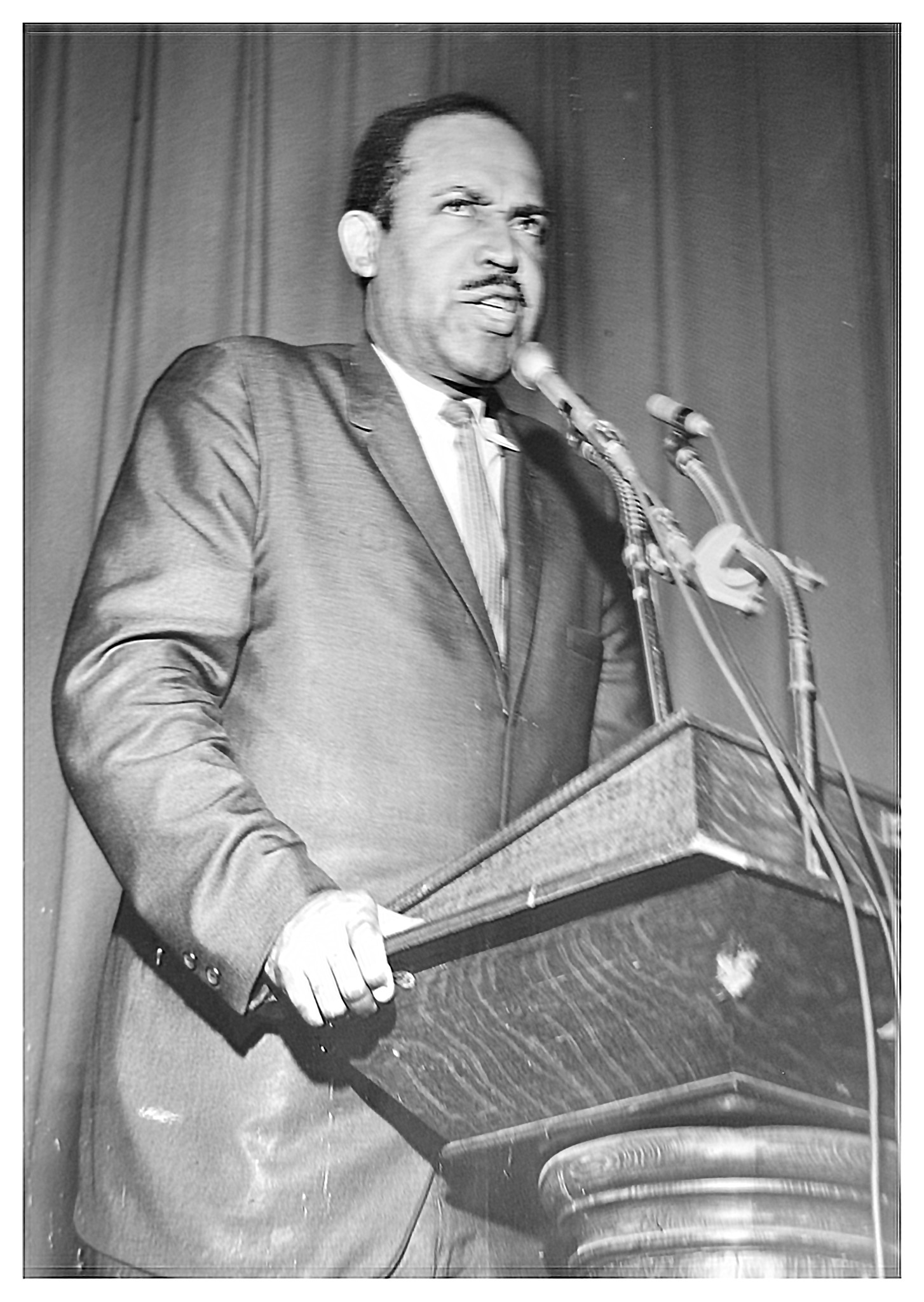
[511,341,636,480]
[646,393,712,436]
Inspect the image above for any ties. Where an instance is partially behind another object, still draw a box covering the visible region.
[439,398,507,678]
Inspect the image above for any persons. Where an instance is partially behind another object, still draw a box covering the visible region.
[49,94,654,1279]
